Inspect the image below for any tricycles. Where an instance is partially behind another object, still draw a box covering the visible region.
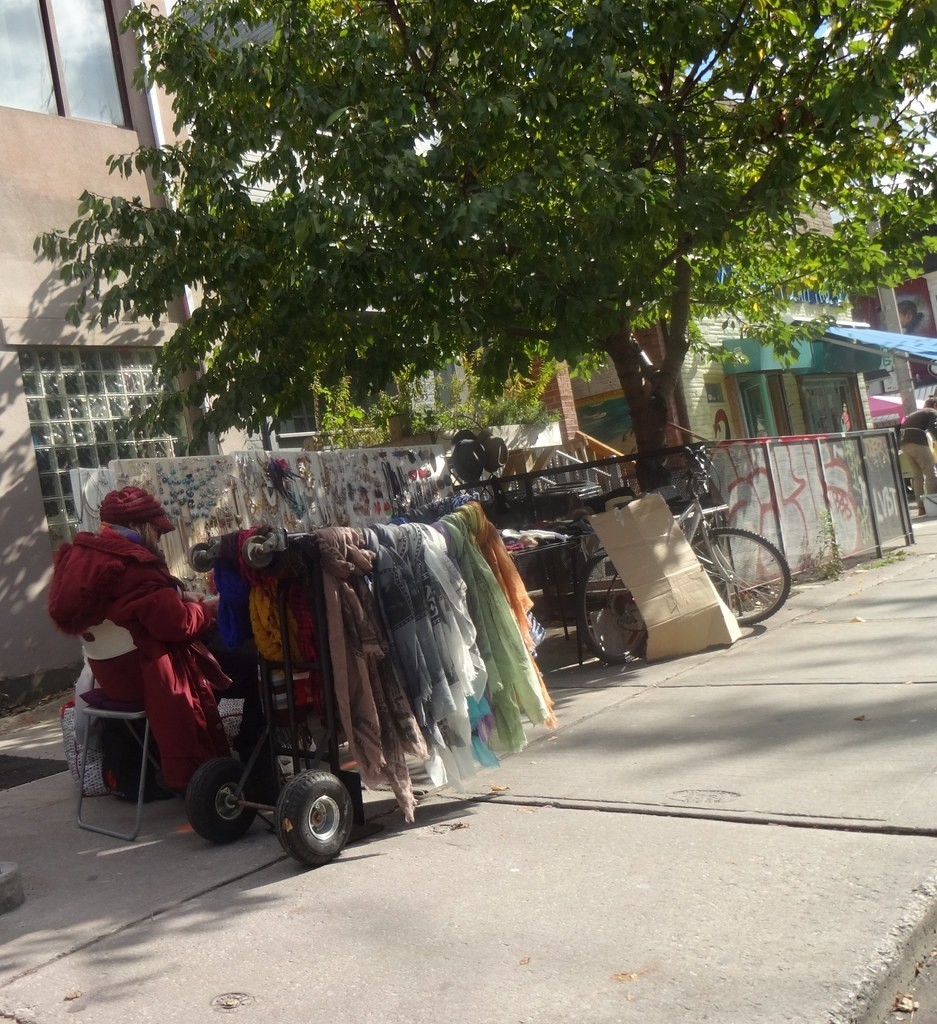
[507,442,791,668]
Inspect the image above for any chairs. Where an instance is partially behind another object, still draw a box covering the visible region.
[76,619,222,842]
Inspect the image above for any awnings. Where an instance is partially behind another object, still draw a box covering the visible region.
[810,323,937,367]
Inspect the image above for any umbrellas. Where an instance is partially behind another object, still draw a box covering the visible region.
[866,394,925,417]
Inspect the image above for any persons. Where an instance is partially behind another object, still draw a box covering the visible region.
[899,395,937,516]
[46,486,286,808]
[896,301,924,335]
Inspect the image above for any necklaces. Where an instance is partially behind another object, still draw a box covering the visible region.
[238,455,284,530]
[379,459,414,519]
[301,454,350,528]
[157,516,191,582]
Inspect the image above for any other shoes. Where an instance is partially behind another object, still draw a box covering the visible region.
[919,507,925,515]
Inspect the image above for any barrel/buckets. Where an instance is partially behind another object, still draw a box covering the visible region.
[920,492,937,522]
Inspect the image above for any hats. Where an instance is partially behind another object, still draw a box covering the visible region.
[99,486,175,535]
[476,430,509,473]
[451,432,486,484]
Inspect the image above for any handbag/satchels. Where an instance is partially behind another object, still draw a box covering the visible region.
[59,699,108,796]
